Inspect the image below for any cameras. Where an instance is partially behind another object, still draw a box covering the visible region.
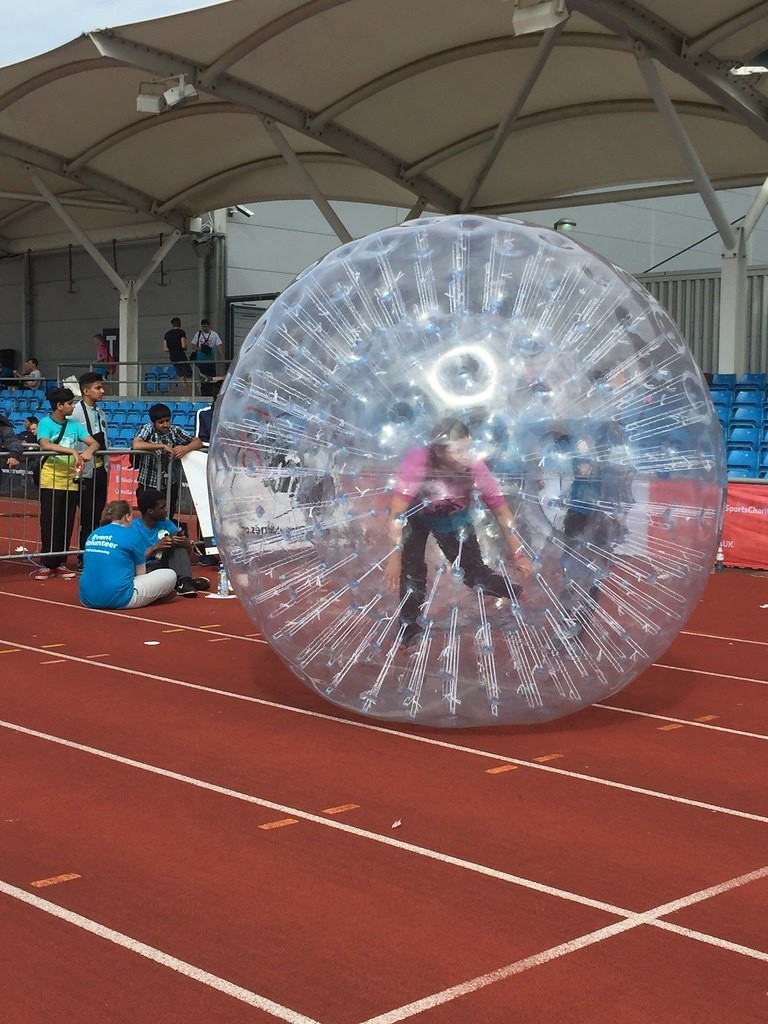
[73,465,83,484]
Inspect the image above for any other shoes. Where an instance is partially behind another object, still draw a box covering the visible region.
[395,624,428,649]
[550,614,592,644]
[507,580,527,602]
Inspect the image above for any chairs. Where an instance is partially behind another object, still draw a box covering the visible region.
[0,361,211,456]
[704,370,768,483]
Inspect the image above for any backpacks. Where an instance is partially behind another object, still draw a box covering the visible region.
[100,345,116,374]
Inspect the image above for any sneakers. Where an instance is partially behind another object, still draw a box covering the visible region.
[176,578,198,596]
[192,577,211,591]
[198,555,220,566]
[55,565,76,577]
[35,566,55,580]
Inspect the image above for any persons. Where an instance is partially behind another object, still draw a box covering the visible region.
[30,421,40,487]
[163,317,193,395]
[0,362,13,390]
[129,489,210,596]
[191,319,222,395]
[193,380,227,570]
[132,403,204,519]
[25,357,41,391]
[92,334,110,396]
[382,416,528,650]
[77,499,178,610]
[13,361,33,390]
[36,388,100,580]
[17,416,32,442]
[68,373,111,578]
[0,414,24,483]
[542,388,634,648]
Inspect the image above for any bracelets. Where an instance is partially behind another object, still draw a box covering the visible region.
[513,551,524,560]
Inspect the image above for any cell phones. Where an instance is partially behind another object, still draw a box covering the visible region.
[177,530,185,537]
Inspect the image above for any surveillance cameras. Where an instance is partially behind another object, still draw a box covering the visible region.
[227,204,255,218]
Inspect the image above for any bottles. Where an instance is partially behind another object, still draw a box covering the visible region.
[73,465,82,484]
[217,564,229,596]
[715,547,724,572]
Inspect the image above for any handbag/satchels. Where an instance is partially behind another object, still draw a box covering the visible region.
[129,445,140,469]
[31,457,43,486]
[190,351,198,367]
[92,431,108,459]
